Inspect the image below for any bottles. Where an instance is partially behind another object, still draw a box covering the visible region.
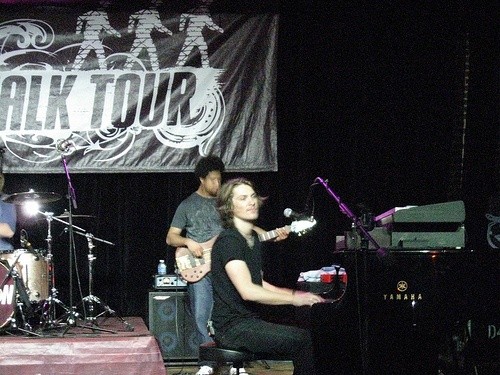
[158,259,166,275]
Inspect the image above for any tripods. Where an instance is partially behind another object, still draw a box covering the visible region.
[0,161,134,338]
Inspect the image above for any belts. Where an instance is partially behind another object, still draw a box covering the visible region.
[0,250,14,254]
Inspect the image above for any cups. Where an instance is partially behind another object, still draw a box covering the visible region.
[336,236,345,250]
[174,262,180,274]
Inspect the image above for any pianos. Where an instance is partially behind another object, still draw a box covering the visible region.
[292,247,500,375]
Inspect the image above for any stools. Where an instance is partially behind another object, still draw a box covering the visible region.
[199,345,250,375]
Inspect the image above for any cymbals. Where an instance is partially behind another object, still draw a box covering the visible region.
[1,191,63,205]
[54,211,97,219]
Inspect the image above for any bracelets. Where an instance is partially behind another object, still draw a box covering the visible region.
[292,289,296,295]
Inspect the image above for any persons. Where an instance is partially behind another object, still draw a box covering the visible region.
[166,155,290,375]
[208,178,325,375]
[0,172,17,253]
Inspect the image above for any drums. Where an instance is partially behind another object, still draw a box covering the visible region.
[0,248,50,306]
[0,262,19,328]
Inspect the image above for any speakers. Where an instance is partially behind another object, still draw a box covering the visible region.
[320,250,500,375]
[148,291,204,360]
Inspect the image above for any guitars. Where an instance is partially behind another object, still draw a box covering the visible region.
[174,217,318,283]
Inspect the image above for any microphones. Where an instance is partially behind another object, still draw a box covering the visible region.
[20,231,25,247]
[284,208,314,222]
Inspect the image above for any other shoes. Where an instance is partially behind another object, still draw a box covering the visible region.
[196,365,214,375]
[230,367,248,375]
